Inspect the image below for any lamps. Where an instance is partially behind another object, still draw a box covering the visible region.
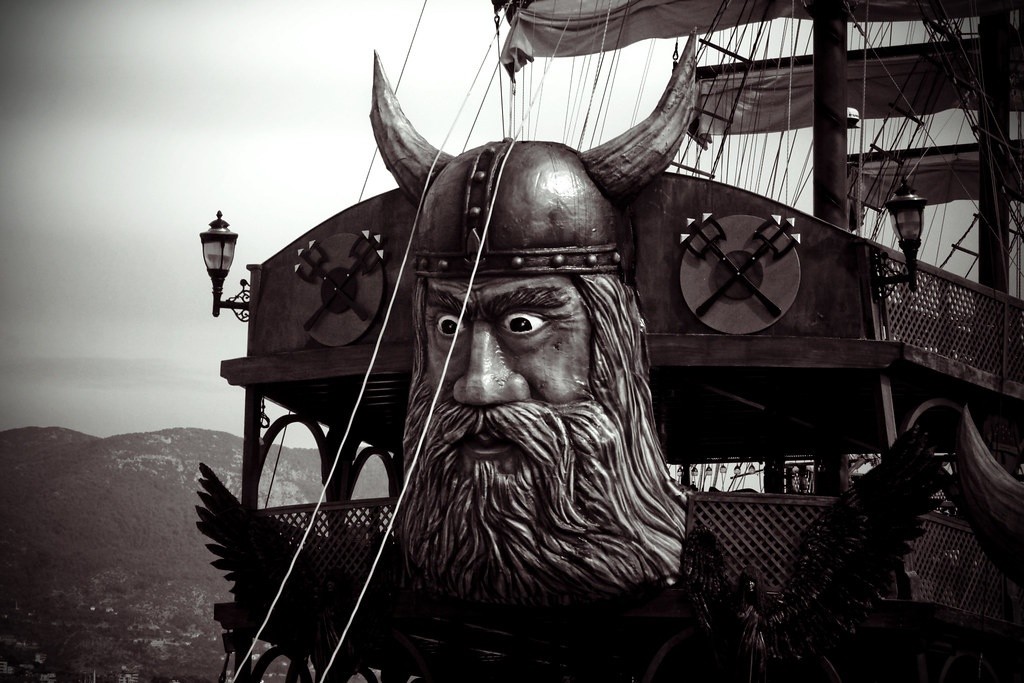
[199,210,249,317]
[869,175,928,304]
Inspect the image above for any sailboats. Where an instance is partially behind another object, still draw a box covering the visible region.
[195,0,1024,683]
